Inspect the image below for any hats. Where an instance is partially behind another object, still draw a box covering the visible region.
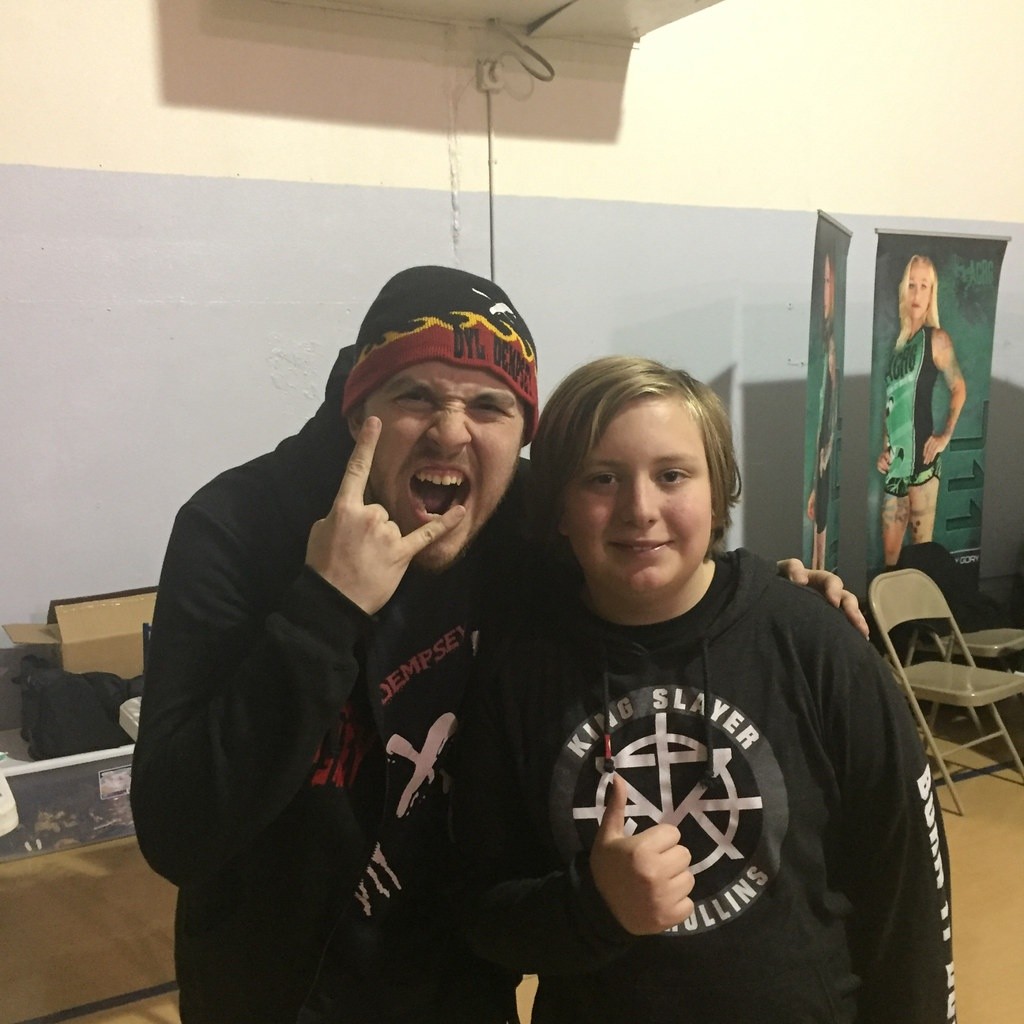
[340,265,540,447]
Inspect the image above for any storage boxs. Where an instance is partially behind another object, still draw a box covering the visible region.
[1,585,158,682]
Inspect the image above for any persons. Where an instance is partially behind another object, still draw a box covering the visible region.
[807,250,839,570]
[131,267,872,1022]
[446,354,957,1023]
[877,250,969,569]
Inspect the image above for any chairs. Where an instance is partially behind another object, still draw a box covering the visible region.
[868,542,1024,815]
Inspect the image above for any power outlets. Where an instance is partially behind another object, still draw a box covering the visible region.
[478,61,503,92]
